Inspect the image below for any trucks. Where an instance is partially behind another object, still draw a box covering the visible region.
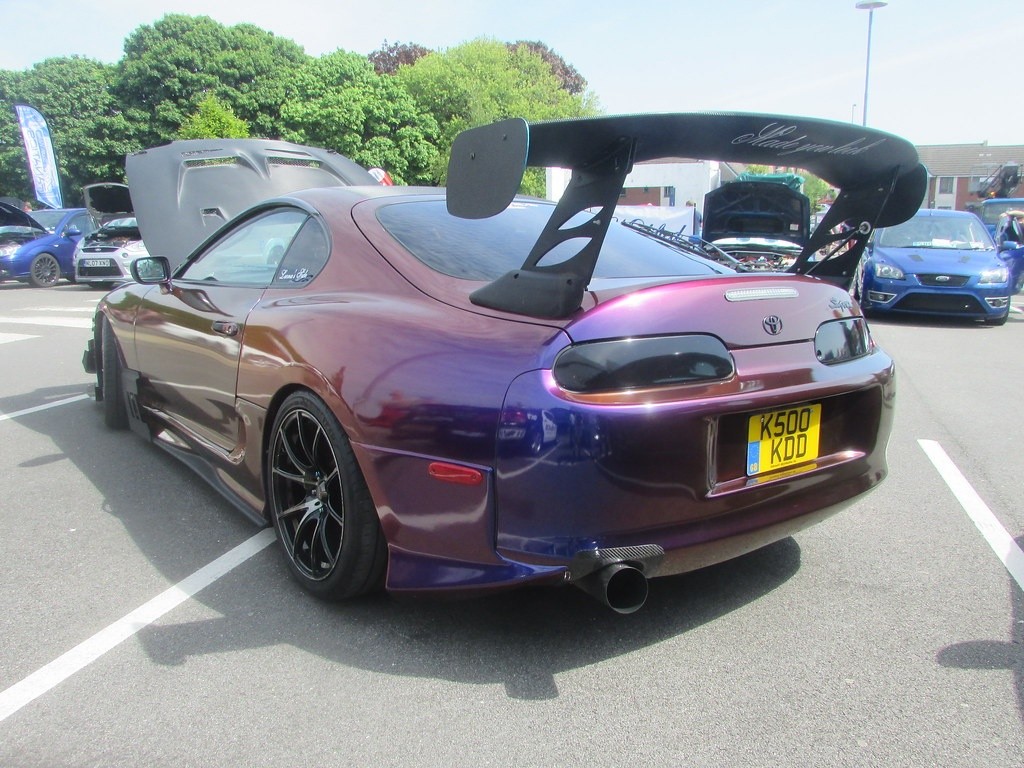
[979,198,1024,242]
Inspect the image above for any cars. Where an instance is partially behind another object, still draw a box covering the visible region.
[0,199,96,286]
[852,209,1024,326]
[699,180,827,274]
[73,181,151,289]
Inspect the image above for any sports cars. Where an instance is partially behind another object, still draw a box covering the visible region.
[81,112,927,616]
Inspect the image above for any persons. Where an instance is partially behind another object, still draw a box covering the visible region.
[24,202,32,211]
[686,199,703,236]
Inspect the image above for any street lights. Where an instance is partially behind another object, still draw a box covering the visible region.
[851,104,857,123]
[856,0,888,127]
